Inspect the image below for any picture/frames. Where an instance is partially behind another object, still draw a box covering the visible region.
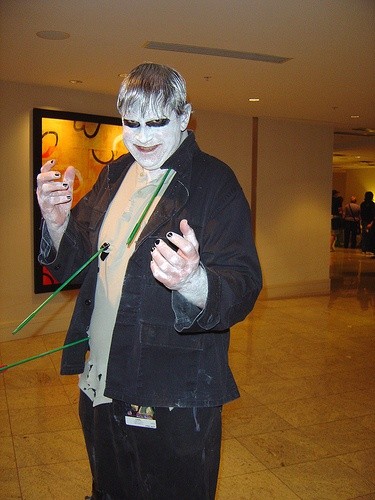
[33,107,123,294]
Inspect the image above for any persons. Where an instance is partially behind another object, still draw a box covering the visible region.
[330,189,375,256]
[35,60,263,500]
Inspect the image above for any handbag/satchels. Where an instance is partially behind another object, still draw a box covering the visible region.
[355,221,361,235]
